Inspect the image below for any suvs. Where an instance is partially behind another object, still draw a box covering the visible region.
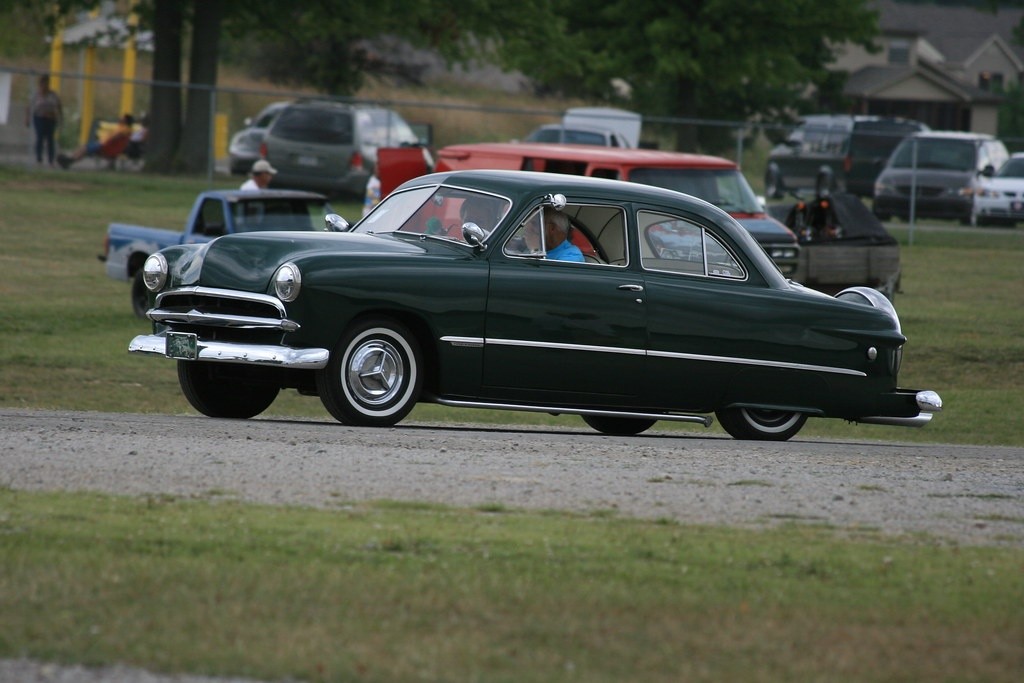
[261,95,427,200]
[373,136,806,294]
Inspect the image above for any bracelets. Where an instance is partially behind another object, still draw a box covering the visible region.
[531,249,539,252]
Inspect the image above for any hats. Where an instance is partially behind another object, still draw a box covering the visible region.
[253,159,277,174]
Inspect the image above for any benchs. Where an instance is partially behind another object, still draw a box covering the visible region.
[92,154,150,172]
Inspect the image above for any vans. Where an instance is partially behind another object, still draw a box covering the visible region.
[763,113,933,201]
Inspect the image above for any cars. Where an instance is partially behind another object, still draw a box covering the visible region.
[763,195,902,306]
[873,130,1011,226]
[970,152,1024,226]
[522,125,630,151]
[127,167,943,443]
[228,98,291,175]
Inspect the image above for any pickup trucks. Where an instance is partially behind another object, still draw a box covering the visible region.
[96,190,344,319]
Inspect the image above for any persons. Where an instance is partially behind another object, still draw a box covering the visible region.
[234,159,278,221]
[363,164,381,217]
[25,72,150,173]
[459,197,498,240]
[509,206,584,263]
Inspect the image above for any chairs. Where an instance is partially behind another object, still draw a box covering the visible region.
[583,253,607,264]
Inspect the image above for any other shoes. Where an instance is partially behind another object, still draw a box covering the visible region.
[57,154,70,168]
